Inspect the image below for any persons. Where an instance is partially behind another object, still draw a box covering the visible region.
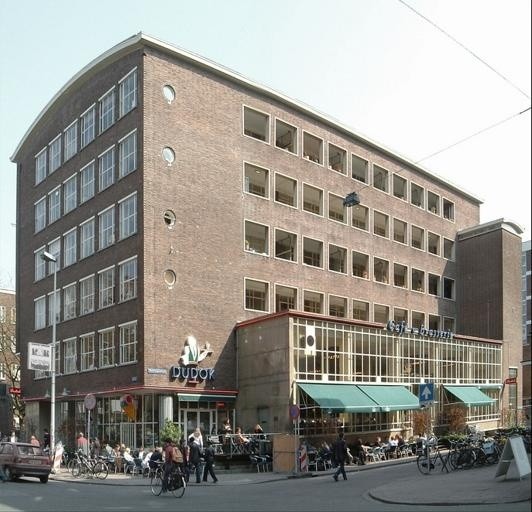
[1,433,9,442]
[9,431,19,443]
[77,428,219,493]
[235,427,253,453]
[301,435,405,471]
[255,424,265,440]
[43,428,49,448]
[417,432,426,444]
[30,436,39,445]
[221,418,232,453]
[332,433,348,481]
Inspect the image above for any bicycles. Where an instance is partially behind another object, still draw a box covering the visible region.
[68,448,109,480]
[149,459,186,497]
[418,426,532,473]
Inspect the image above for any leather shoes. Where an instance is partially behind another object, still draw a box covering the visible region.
[333,476,347,481]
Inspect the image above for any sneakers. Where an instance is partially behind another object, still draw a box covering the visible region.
[196,479,218,483]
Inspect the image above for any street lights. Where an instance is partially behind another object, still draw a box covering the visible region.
[40,252,57,462]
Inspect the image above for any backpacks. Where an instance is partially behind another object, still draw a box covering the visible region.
[172,447,183,463]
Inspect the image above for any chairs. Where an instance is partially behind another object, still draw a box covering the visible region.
[306,436,423,471]
[99,434,272,477]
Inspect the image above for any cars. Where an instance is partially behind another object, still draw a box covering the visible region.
[0,441,52,484]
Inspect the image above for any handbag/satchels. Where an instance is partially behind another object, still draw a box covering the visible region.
[198,449,203,456]
